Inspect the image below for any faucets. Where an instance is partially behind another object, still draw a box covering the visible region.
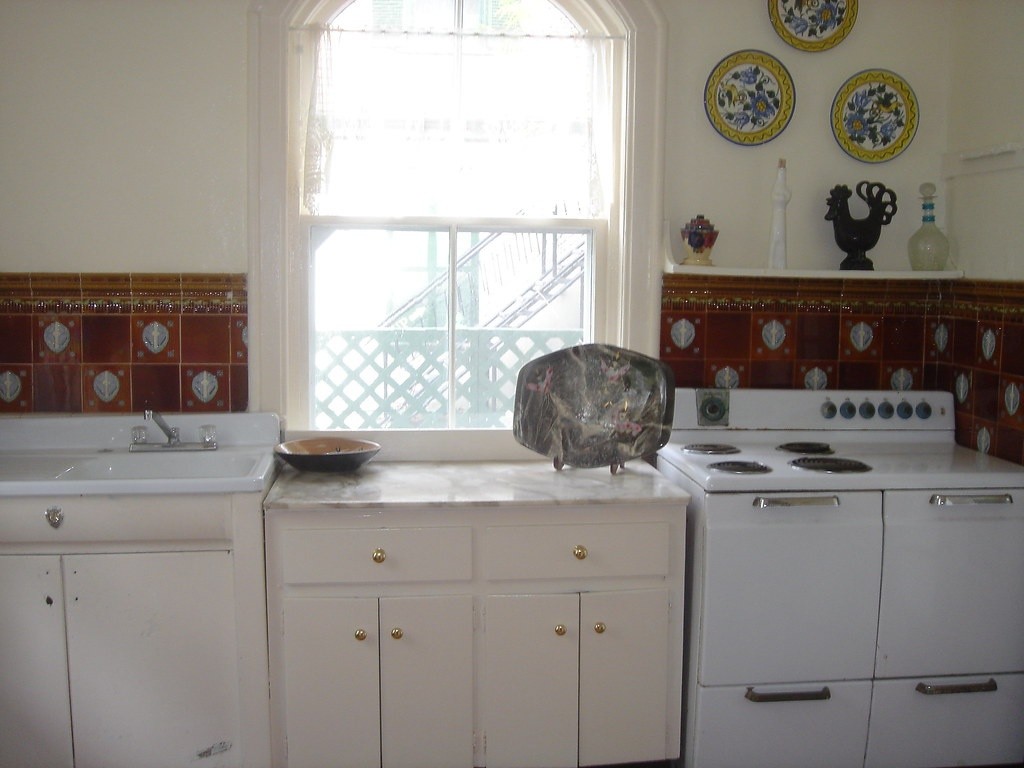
[143,408,181,445]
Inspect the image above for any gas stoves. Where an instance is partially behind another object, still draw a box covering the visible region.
[656,386,1024,490]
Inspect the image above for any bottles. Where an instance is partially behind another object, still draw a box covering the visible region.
[769,167,792,270]
[906,183,949,271]
[680,215,719,266]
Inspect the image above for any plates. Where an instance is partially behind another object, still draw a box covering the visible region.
[511,343,675,468]
[829,68,921,165]
[702,48,798,147]
[768,0,859,52]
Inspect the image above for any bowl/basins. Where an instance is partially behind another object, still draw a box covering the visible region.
[274,437,382,473]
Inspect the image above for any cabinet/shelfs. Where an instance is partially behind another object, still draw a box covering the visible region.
[264,507,474,768]
[480,510,672,768]
[1,541,242,767]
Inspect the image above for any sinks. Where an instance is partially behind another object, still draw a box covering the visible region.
[53,448,263,482]
